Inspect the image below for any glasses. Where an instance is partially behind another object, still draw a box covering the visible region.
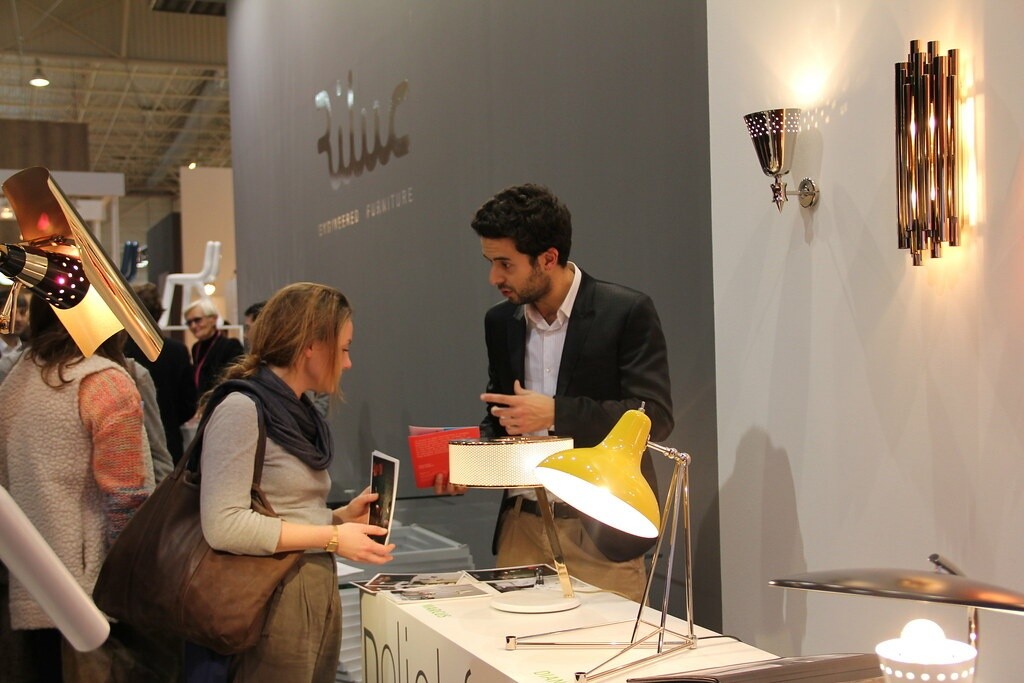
[185,316,206,326]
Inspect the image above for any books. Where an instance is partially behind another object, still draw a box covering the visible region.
[408,425,480,489]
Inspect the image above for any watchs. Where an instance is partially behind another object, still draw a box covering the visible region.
[323,526,339,552]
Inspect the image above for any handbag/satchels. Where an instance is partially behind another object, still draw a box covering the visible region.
[90,390,304,654]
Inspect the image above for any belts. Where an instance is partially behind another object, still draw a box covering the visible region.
[508,496,579,519]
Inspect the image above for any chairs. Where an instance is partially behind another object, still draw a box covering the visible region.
[158,241,223,330]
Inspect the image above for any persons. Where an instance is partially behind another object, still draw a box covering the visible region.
[0,293,30,358]
[184,299,245,411]
[124,283,197,465]
[124,357,174,485]
[433,185,675,607]
[0,290,156,683]
[182,283,395,683]
[243,301,269,348]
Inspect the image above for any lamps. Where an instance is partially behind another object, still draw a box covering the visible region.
[505,401,698,683]
[0,166,165,362]
[448,436,582,613]
[770,553,1023,683]
[29,58,49,87]
[744,108,821,210]
[896,40,960,266]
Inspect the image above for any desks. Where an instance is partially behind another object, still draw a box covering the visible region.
[360,564,781,683]
